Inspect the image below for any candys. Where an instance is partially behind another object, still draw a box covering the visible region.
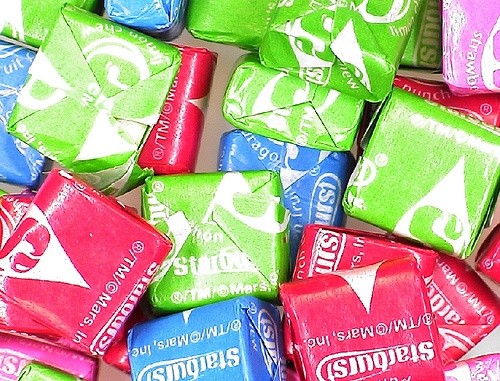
[0,0,500,381]
